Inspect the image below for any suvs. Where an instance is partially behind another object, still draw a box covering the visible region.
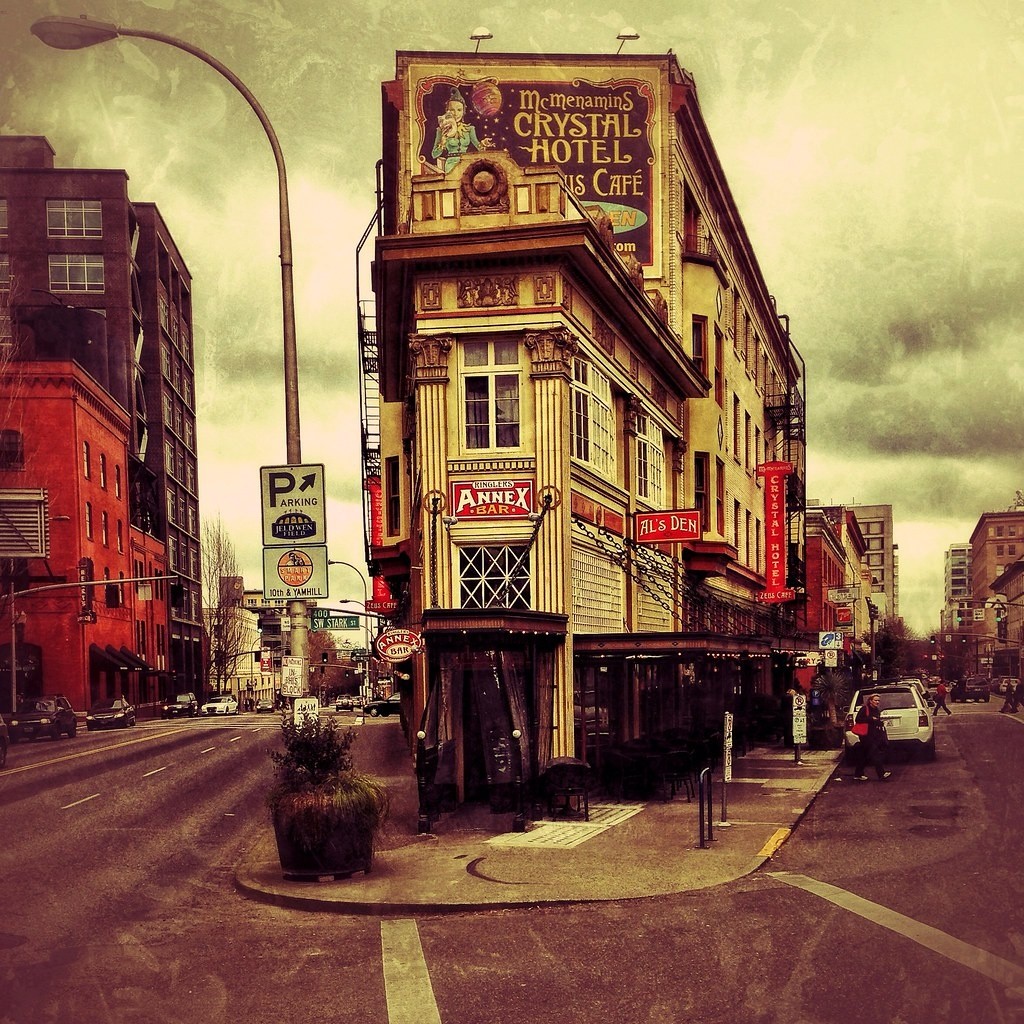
[336,693,355,712]
[950,676,991,702]
[841,683,936,763]
[7,695,78,741]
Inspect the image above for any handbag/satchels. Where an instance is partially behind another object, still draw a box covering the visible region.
[852,705,869,737]
[933,695,941,703]
[872,725,888,745]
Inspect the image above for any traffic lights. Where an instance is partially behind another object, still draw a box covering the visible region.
[997,608,1001,621]
[322,653,327,662]
[874,606,879,619]
[957,609,962,622]
[869,603,876,618]
[961,635,967,644]
[930,636,936,645]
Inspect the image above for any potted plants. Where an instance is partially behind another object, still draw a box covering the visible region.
[263,702,390,875]
[806,674,848,749]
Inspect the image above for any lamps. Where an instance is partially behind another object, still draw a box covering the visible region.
[527,511,540,527]
[442,515,458,531]
[468,26,493,52]
[616,27,639,54]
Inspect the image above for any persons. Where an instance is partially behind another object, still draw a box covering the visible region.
[781,688,798,744]
[853,694,892,781]
[243,697,255,712]
[275,698,286,710]
[931,680,953,717]
[999,680,1019,713]
[1009,678,1024,713]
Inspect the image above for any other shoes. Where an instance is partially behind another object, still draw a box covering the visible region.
[853,775,868,780]
[880,772,892,779]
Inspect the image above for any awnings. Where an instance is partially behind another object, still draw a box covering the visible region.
[106,644,143,670]
[89,642,128,670]
[120,646,154,671]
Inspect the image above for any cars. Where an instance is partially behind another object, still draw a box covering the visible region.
[200,696,239,716]
[351,696,361,709]
[159,692,199,717]
[948,679,957,688]
[85,697,136,729]
[987,675,1020,693]
[362,691,401,717]
[891,673,928,699]
[256,698,276,713]
[927,676,941,686]
[0,711,10,768]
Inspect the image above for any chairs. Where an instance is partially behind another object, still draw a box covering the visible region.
[540,755,591,822]
[732,701,786,759]
[601,720,724,803]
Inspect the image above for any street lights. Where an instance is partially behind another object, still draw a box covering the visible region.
[339,599,373,650]
[328,560,371,681]
[10,514,70,712]
[28,16,313,739]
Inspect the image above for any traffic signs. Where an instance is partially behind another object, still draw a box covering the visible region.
[259,463,327,546]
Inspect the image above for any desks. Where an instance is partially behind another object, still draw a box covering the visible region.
[619,739,689,790]
